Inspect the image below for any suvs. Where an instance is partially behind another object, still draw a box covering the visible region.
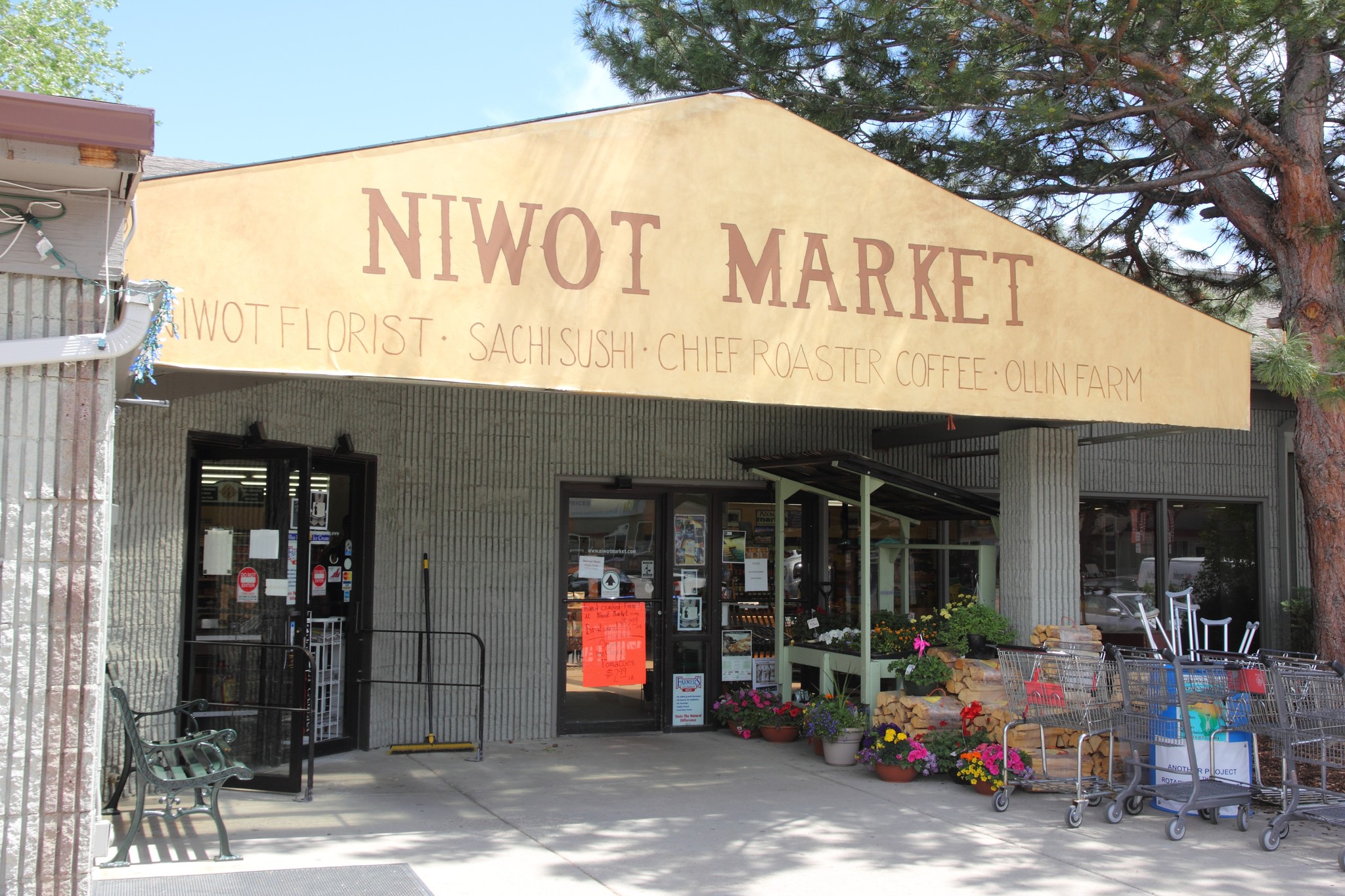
[1079,589,1163,635]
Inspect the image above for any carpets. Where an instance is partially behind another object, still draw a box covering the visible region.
[90,863,436,896]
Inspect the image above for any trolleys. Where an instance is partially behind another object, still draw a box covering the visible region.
[986,638,1343,866]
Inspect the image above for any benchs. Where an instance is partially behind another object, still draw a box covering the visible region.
[100,661,254,867]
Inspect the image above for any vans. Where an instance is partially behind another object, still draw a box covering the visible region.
[1136,554,1247,598]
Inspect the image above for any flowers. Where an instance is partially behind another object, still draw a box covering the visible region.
[711,592,1035,796]
